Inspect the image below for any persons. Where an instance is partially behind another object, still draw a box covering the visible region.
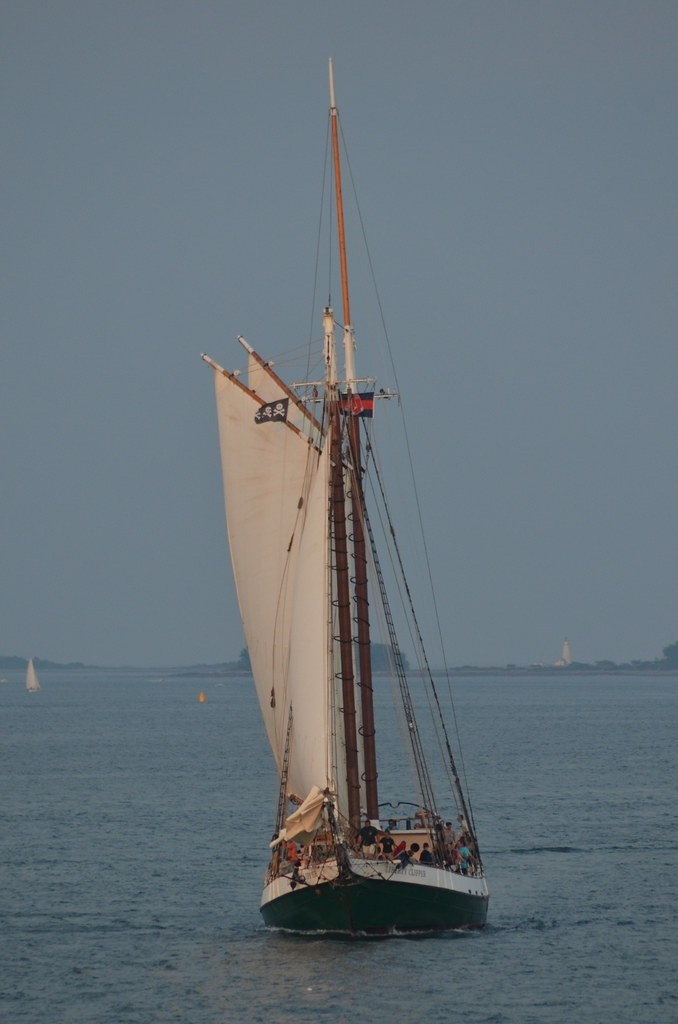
[385,819,399,832]
[379,830,395,860]
[359,820,380,860]
[420,842,434,866]
[392,841,418,863]
[444,815,483,876]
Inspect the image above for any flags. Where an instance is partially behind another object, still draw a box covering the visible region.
[338,391,374,418]
[254,397,289,424]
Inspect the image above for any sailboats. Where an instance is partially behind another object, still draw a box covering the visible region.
[199,58,490,931]
[25,657,43,693]
